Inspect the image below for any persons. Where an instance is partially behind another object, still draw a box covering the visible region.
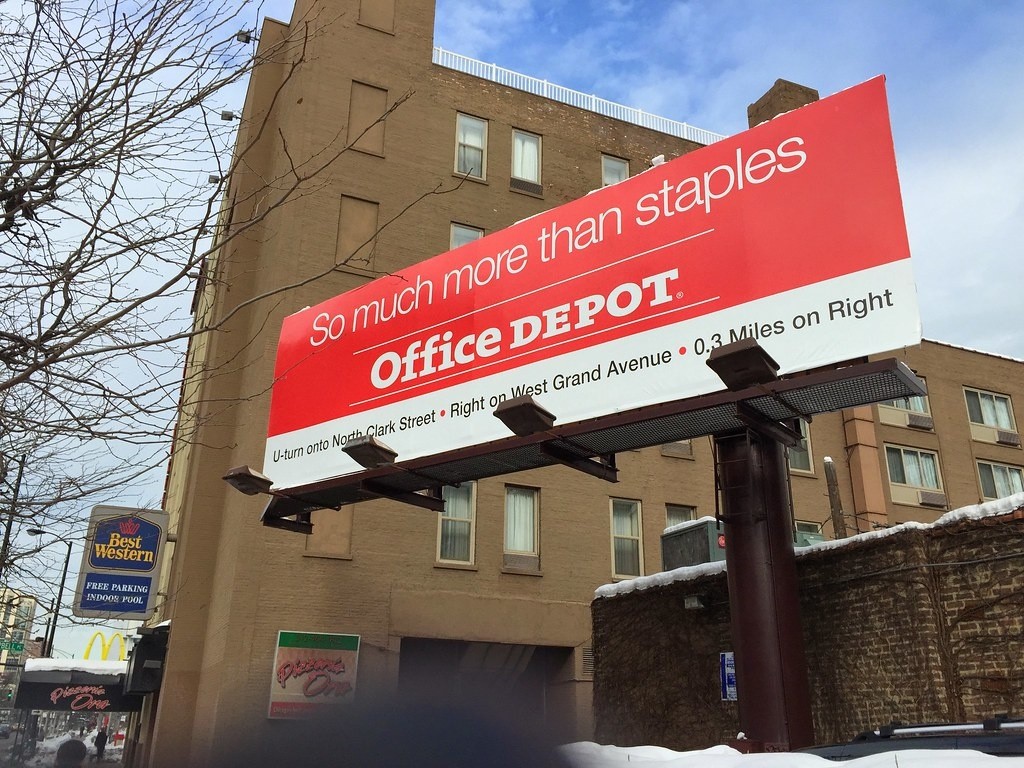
[79,724,85,738]
[93,726,108,762]
[54,739,87,768]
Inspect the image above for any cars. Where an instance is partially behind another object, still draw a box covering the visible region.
[0,724,10,738]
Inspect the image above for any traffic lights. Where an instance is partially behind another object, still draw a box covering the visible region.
[8,691,13,701]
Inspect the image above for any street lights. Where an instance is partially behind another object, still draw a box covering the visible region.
[25,527,73,660]
[35,637,53,659]
